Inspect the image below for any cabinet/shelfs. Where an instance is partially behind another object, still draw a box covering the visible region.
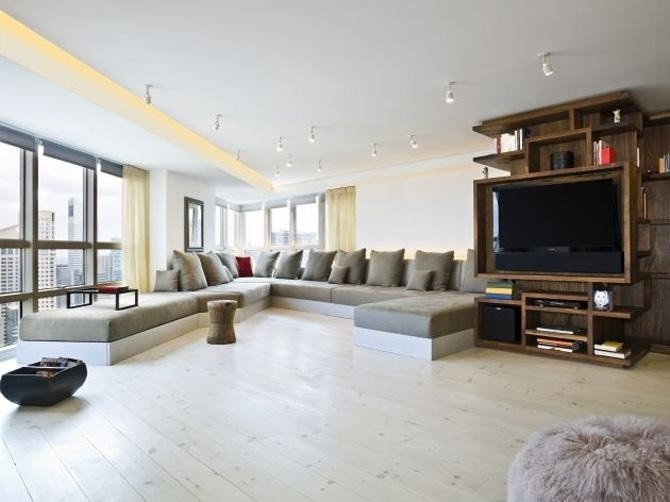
[472,89,651,370]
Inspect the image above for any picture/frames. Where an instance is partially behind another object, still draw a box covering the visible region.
[184,195,204,252]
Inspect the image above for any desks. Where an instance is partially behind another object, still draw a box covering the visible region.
[66,287,138,310]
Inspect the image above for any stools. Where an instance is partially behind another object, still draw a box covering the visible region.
[207,300,237,344]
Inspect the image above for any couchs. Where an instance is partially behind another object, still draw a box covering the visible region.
[19,261,477,367]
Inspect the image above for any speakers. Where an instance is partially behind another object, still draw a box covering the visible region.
[481,305,520,344]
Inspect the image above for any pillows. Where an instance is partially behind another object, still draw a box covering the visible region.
[405,270,433,291]
[254,251,280,277]
[172,250,207,291]
[197,251,230,286]
[154,270,179,292]
[273,250,303,279]
[460,249,506,294]
[326,248,366,285]
[236,257,253,277]
[327,266,349,284]
[302,248,337,281]
[365,249,404,287]
[216,252,238,278]
[415,250,454,293]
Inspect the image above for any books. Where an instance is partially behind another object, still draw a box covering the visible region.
[535,339,581,353]
[99,283,128,292]
[594,342,631,359]
[492,128,529,153]
[538,322,586,335]
[593,141,615,165]
[485,281,522,299]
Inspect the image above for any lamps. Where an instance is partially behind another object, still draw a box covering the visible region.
[308,124,316,141]
[144,83,153,103]
[213,113,221,129]
[408,127,418,150]
[446,80,456,104]
[537,51,555,77]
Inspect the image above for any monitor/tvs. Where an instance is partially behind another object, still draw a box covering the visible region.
[492,176,625,275]
